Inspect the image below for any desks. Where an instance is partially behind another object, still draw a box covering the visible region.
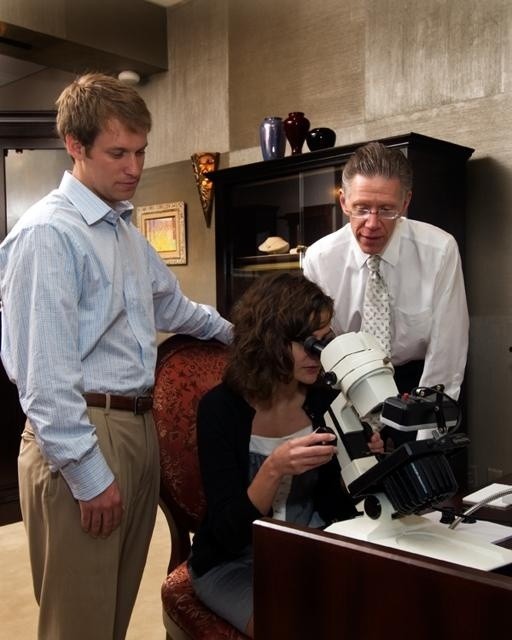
[252,474,512,640]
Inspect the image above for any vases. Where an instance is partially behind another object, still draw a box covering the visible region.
[259,112,336,161]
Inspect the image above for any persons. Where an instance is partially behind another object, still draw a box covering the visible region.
[2,67,234,640]
[303,139,474,402]
[184,271,388,640]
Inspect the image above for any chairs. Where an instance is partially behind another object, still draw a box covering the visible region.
[150,336,253,640]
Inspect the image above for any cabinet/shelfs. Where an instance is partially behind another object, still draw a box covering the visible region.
[204,134,475,322]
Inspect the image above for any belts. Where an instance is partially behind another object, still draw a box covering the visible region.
[83,393,154,416]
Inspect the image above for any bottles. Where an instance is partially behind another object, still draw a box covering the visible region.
[260,112,336,161]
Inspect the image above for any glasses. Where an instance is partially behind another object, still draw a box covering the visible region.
[344,197,405,220]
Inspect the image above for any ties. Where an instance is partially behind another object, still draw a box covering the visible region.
[364,257,391,352]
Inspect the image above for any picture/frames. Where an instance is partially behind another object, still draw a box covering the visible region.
[136,201,187,265]
